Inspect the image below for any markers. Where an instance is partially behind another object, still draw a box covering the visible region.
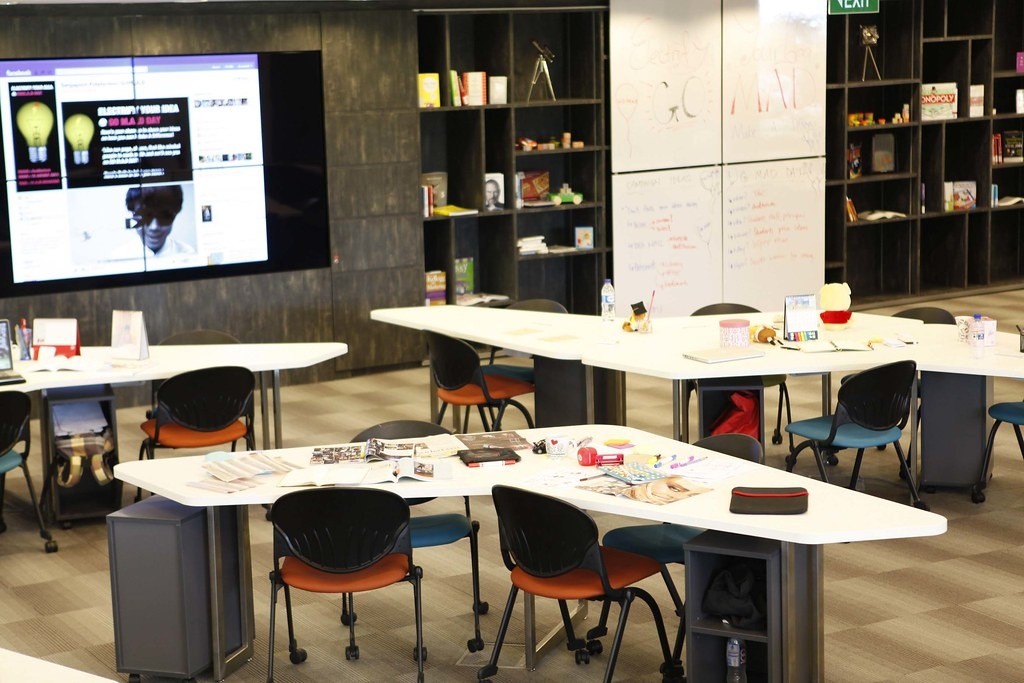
[468,460,516,467]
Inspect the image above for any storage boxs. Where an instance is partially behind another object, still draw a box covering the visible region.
[955,315,997,349]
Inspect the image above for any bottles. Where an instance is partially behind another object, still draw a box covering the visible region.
[969,314,986,362]
[600,276,617,324]
[563,132,572,149]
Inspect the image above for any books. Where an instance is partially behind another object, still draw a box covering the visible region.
[799,336,872,353]
[845,196,907,222]
[684,347,763,364]
[418,71,548,308]
[922,83,1024,211]
[278,438,438,486]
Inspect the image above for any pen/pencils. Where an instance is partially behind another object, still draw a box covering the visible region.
[670,456,694,469]
[1015,324,1022,334]
[647,290,656,319]
[17,318,32,360]
[654,454,677,468]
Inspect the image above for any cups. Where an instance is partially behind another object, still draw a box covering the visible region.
[15,327,32,359]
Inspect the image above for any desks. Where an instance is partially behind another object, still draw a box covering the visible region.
[370,305,630,427]
[115,425,949,683]
[581,311,1024,494]
[0,343,349,531]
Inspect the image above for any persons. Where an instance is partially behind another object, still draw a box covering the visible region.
[101,184,194,271]
[484,179,505,210]
[621,476,712,507]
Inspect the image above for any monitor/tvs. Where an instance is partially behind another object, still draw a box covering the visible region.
[0,50,332,298]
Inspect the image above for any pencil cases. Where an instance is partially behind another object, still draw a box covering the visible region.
[457,447,521,466]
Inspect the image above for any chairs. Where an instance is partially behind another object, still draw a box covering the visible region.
[0,297,1024,683]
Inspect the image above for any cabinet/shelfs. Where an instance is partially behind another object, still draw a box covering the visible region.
[411,4,609,354]
[824,0,1024,311]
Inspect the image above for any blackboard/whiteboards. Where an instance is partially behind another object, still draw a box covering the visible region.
[608,1,829,317]
